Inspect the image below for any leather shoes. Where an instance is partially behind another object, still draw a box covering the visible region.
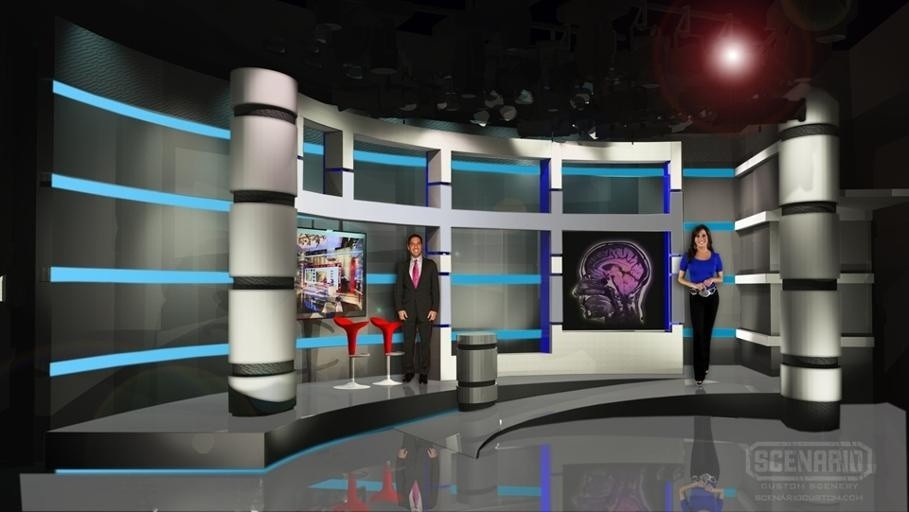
[419,374,427,384]
[403,373,414,382]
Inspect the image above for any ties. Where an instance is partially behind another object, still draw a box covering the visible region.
[413,260,419,288]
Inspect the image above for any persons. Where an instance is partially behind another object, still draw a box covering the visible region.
[678,387,724,512]
[678,224,724,386]
[395,385,440,512]
[393,234,440,385]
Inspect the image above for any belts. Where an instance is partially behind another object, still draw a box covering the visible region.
[688,286,716,297]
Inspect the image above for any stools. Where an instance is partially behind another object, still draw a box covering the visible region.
[371,470,406,503]
[369,316,406,386]
[334,478,368,512]
[333,315,372,390]
